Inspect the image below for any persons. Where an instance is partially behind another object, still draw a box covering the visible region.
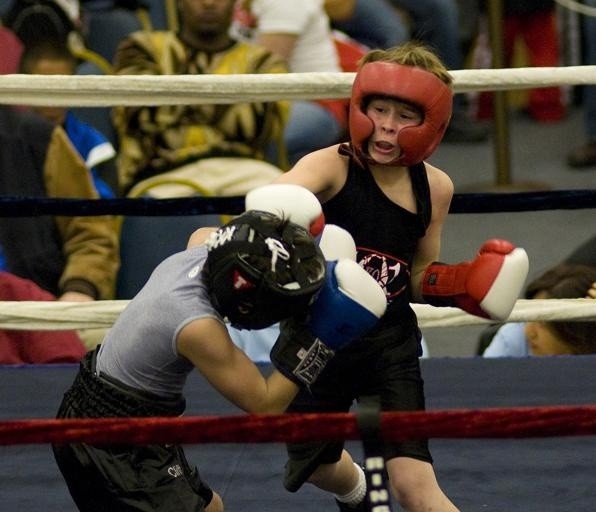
[245,40,531,511]
[51,211,388,512]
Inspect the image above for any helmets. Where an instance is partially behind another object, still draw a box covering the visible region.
[349,62,453,166]
[205,210,326,329]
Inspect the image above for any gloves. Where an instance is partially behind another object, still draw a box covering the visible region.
[245,183,325,237]
[269,224,387,389]
[422,239,530,322]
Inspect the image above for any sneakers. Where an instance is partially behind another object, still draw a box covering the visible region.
[441,110,487,142]
[568,144,596,166]
